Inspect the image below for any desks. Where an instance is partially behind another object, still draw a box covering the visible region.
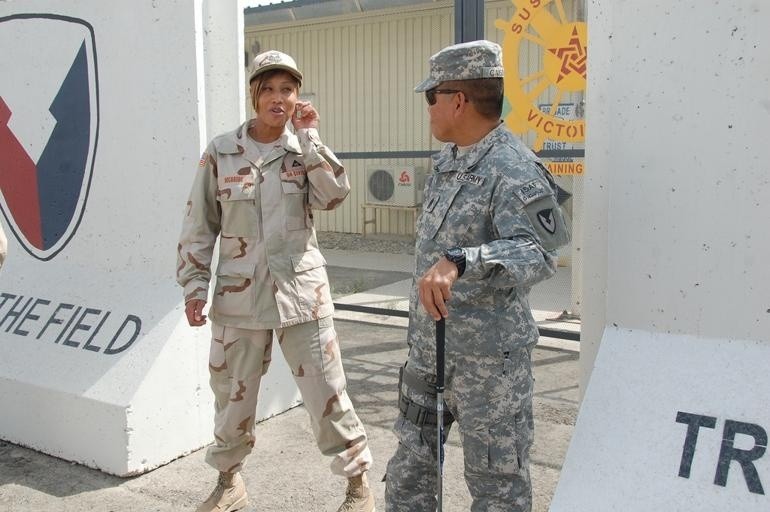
[359,204,422,241]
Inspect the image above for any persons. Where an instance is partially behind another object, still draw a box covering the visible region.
[381,40,569,511]
[177,49,375,510]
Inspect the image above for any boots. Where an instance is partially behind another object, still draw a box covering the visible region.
[196,471,248,512]
[336,473,376,512]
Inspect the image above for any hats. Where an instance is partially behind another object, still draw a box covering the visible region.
[249,50,302,85]
[414,40,504,93]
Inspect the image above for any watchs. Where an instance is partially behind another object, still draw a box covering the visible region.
[444,247,467,277]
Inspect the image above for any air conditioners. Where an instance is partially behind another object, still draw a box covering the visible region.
[365,165,427,205]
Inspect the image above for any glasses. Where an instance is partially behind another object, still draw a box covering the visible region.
[425,90,469,105]
